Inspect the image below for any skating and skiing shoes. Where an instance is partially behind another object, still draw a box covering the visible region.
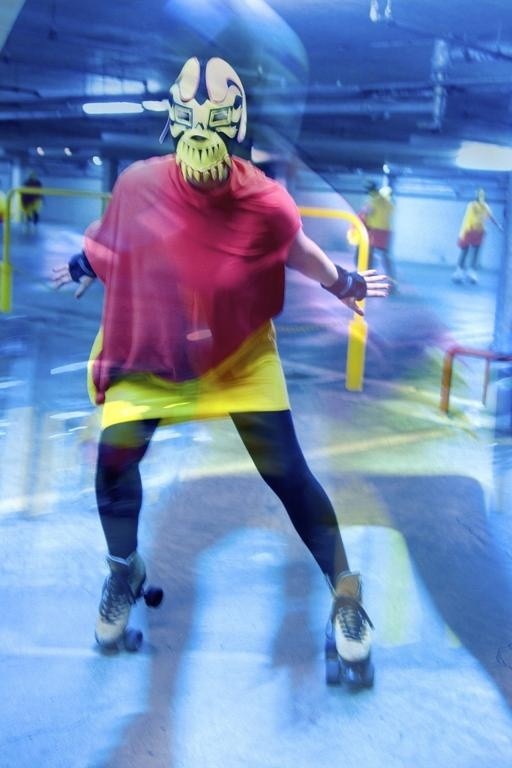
[321,569,377,694]
[93,550,165,653]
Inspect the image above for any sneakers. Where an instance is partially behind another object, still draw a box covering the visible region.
[452,267,479,285]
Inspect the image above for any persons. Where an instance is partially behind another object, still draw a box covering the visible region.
[44,51,399,672]
[18,171,44,223]
[353,179,394,280]
[448,186,505,284]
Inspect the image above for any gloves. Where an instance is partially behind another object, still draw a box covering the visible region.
[68,250,97,283]
[319,263,366,301]
